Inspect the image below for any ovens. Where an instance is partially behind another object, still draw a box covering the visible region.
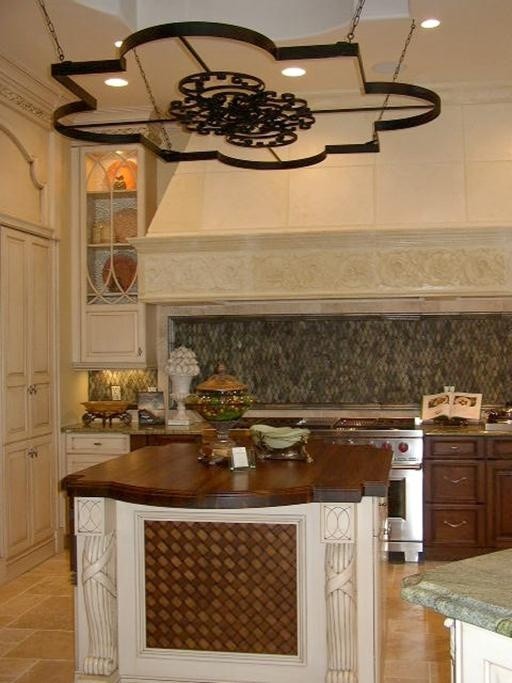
[328,437,423,542]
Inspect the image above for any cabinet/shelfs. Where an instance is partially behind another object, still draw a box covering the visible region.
[68,142,155,372]
[64,433,147,478]
[424,435,511,560]
[0,225,68,581]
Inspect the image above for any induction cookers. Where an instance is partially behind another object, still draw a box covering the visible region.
[333,417,415,430]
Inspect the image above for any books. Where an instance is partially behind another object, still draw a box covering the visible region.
[420,391,482,420]
[136,388,166,427]
